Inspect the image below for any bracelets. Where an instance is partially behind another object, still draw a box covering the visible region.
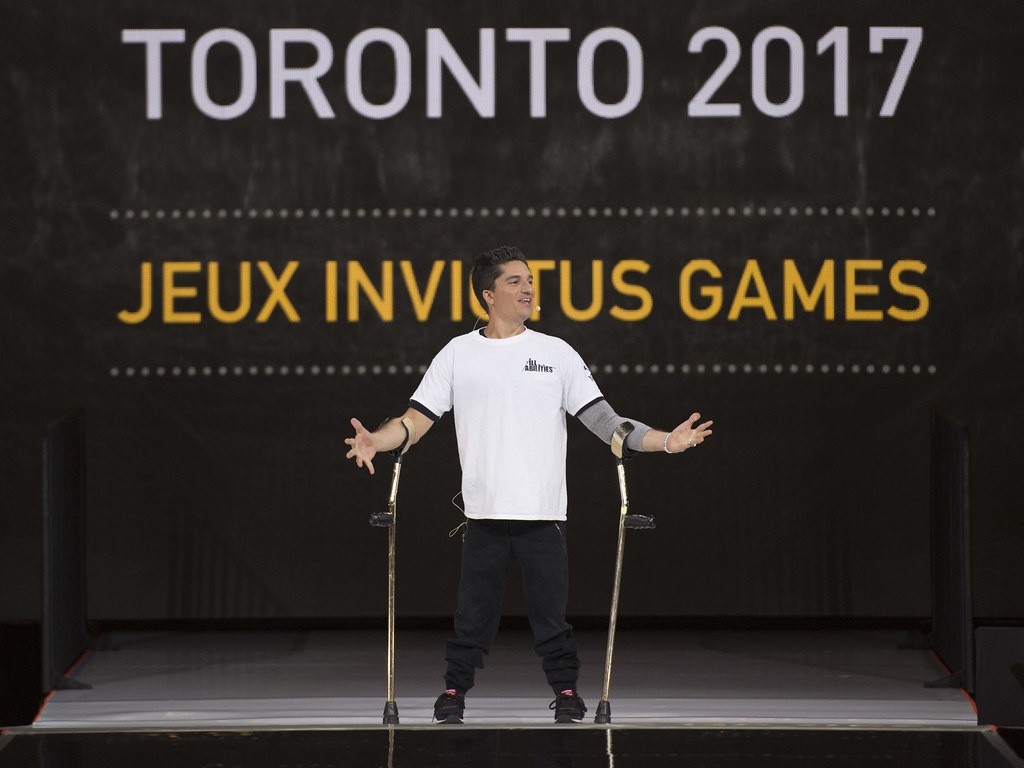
[663,433,672,453]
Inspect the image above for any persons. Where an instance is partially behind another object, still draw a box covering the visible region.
[343,248,713,724]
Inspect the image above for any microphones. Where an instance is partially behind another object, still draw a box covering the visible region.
[536,306,541,311]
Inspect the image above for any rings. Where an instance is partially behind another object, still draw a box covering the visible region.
[688,440,696,446]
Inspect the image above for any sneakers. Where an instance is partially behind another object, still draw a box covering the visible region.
[432,689,465,723]
[549,689,587,722]
